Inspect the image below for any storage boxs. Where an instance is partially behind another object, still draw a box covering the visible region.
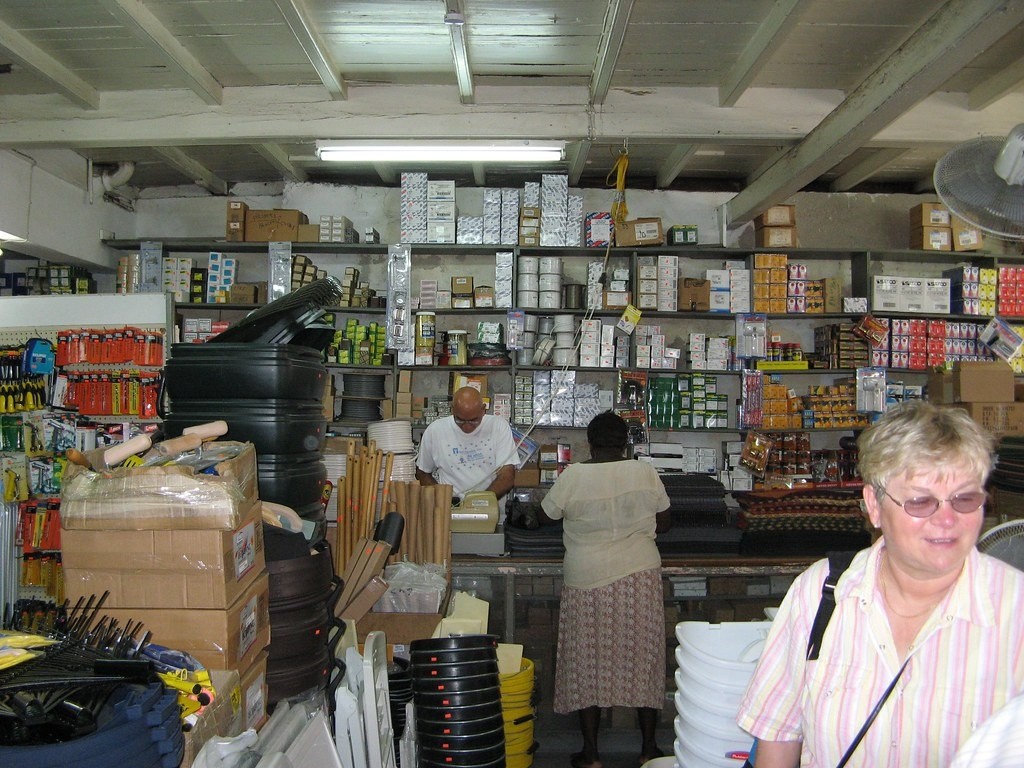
[60,171,1024,768]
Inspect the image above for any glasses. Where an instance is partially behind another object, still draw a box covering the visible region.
[453,408,482,425]
[876,483,987,519]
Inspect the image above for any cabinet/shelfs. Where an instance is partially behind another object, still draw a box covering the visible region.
[107,236,1024,500]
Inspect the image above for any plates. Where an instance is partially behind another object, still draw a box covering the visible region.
[319,454,348,528]
[368,421,416,520]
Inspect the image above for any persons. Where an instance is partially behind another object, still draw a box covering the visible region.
[535,410,674,768]
[734,396,1024,768]
[416,386,521,558]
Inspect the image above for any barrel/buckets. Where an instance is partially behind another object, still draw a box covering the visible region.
[388,633,502,768]
[499,656,540,768]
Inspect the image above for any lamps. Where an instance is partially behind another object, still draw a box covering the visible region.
[444,11,465,26]
[316,136,567,164]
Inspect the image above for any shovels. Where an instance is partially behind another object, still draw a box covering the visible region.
[372,512,405,579]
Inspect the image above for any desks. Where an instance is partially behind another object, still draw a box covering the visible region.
[451,555,828,646]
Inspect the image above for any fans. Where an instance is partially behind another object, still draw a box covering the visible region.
[933,124,1024,239]
[976,519,1024,572]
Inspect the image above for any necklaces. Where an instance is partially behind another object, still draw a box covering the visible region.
[876,549,950,621]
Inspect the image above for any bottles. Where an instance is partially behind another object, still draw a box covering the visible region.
[447,330,467,365]
[416,312,435,346]
[768,342,802,361]
